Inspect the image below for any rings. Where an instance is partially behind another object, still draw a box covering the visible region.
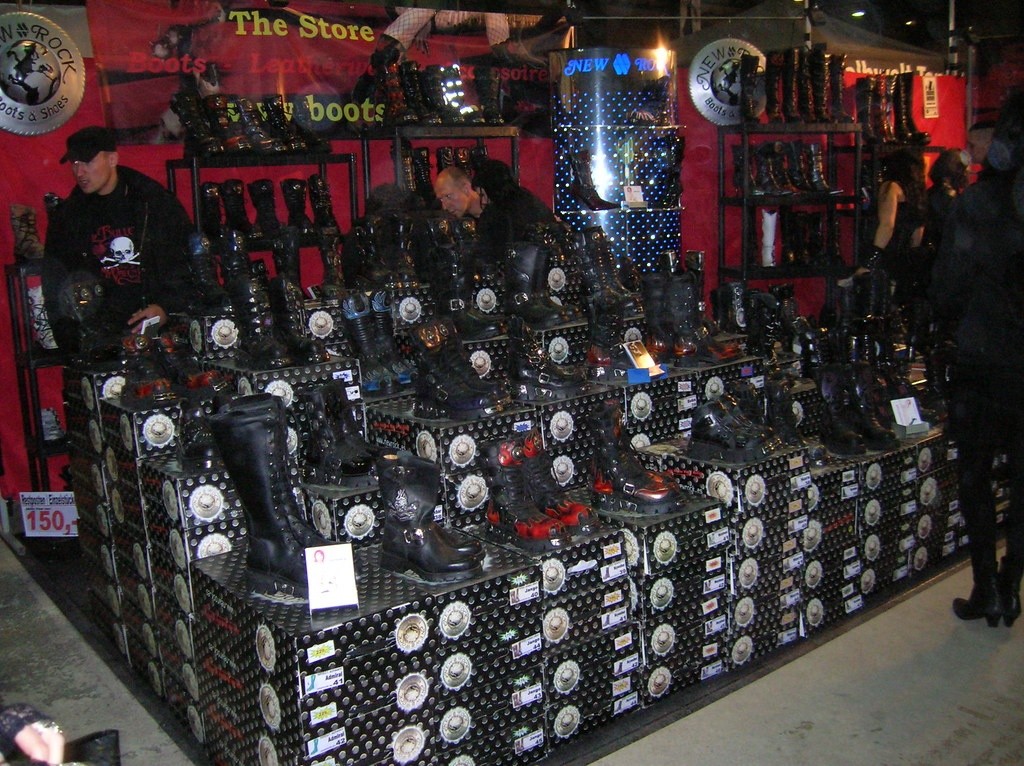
[45,719,64,734]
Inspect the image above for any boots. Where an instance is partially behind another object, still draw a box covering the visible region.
[739,42,856,126]
[348,47,507,131]
[167,87,332,158]
[8,202,47,264]
[953,556,1024,626]
[119,139,952,608]
[26,287,58,352]
[855,69,930,147]
[626,71,676,127]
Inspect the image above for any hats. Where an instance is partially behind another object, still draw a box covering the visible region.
[58,125,117,164]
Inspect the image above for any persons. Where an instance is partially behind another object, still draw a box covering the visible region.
[836,86,1023,628]
[312,550,326,566]
[341,158,556,289]
[41,124,195,357]
[0,702,66,766]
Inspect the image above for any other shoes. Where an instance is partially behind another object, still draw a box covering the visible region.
[42,407,64,445]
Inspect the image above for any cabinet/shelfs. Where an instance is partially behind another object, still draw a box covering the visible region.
[391,127,525,218]
[716,123,942,353]
[5,261,70,492]
[166,153,361,284]
